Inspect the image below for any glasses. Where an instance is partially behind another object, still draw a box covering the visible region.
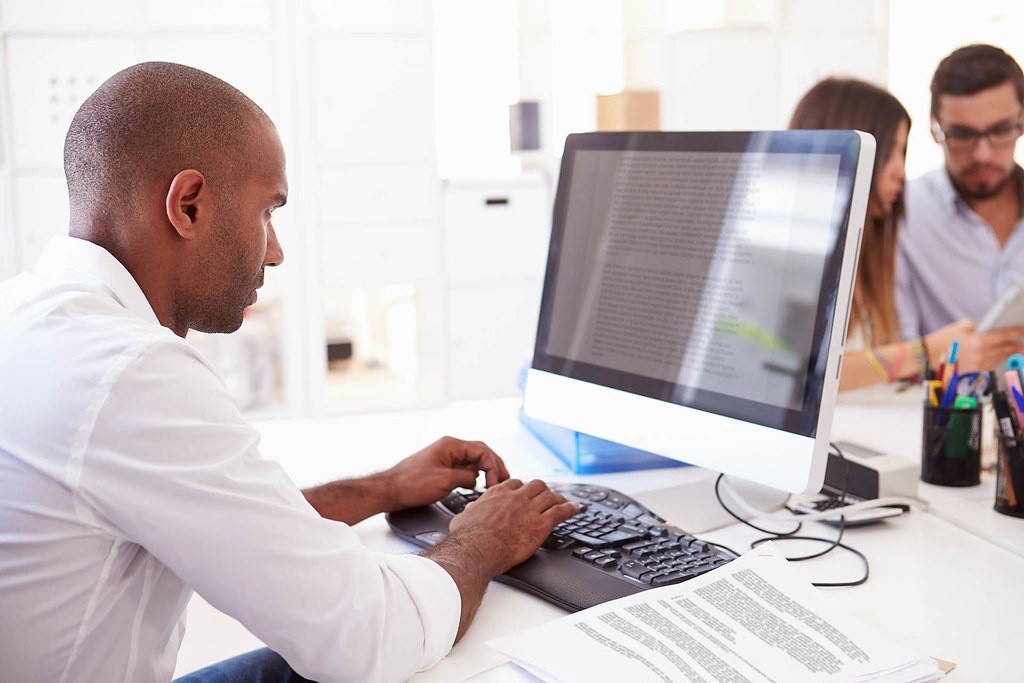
[933,110,1023,154]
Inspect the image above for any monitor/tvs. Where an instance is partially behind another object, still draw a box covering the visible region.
[526,130,876,530]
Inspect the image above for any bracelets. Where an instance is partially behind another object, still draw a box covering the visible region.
[863,340,924,383]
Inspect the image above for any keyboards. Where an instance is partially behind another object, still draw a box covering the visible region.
[387,482,738,613]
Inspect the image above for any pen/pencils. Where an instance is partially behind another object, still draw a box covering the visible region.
[920,340,992,415]
[989,353,1024,437]
[973,284,1022,335]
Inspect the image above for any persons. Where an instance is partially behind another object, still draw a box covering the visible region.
[0,60,579,683]
[892,46,1023,376]
[789,78,1023,392]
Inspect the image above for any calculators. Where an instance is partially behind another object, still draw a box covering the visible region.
[788,493,904,527]
